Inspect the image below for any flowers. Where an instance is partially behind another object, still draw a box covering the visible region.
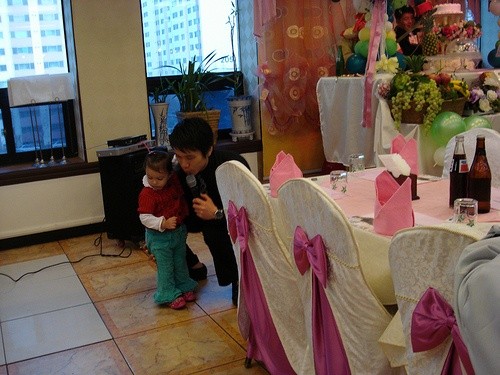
[470,72,500,112]
[374,54,399,74]
[434,20,482,42]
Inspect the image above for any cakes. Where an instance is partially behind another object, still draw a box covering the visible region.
[435,3,462,14]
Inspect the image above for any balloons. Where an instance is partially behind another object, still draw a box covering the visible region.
[429,111,466,148]
[464,116,492,135]
[345,9,406,73]
[489,0,500,17]
[433,146,447,167]
[486,41,500,69]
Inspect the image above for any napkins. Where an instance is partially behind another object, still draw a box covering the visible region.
[391,134,418,176]
[269,150,303,199]
[373,170,415,236]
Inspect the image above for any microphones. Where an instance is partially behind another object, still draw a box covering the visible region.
[185,175,201,199]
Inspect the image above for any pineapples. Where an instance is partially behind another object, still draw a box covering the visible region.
[422,15,438,57]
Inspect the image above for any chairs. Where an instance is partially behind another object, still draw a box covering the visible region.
[276,179,409,375]
[376,224,480,375]
[442,128,500,185]
[216,160,312,375]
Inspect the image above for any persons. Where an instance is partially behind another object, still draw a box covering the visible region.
[392,5,423,55]
[137,148,197,309]
[167,119,255,307]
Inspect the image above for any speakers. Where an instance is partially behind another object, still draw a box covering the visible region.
[97,147,165,243]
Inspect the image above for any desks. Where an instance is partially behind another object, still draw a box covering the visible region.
[316,69,500,172]
[374,103,500,177]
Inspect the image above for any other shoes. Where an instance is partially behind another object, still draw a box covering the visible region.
[183,289,194,301]
[166,296,186,310]
[231,280,239,305]
[189,263,207,281]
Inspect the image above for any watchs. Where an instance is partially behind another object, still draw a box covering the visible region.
[215,208,224,220]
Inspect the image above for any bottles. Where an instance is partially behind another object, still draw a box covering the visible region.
[449,135,469,208]
[335,45,344,76]
[468,134,491,214]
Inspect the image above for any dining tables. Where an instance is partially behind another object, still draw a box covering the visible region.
[258,163,500,322]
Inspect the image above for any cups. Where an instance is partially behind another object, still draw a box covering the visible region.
[454,198,478,231]
[329,170,347,197]
[349,152,365,177]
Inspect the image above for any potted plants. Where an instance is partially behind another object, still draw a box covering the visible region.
[162,49,229,146]
[223,67,256,142]
[147,73,171,146]
